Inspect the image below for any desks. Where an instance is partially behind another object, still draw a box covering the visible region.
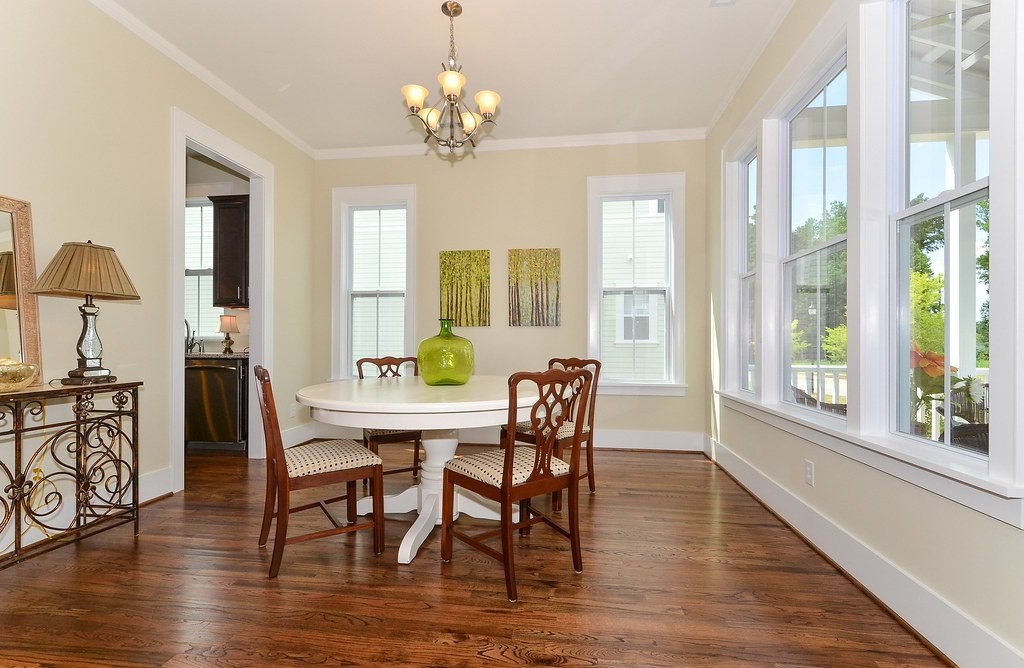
[295,375,572,564]
[1,382,144,569]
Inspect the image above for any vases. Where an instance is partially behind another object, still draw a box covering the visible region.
[417,318,475,386]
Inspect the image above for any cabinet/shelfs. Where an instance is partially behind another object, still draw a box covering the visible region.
[186,358,249,450]
[207,195,249,311]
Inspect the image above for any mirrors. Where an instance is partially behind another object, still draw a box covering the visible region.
[0,195,44,387]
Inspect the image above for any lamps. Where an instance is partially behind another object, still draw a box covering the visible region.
[28,240,141,386]
[0,251,18,310]
[400,1,501,153]
[215,315,240,353]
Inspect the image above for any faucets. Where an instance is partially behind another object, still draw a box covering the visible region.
[184,319,205,354]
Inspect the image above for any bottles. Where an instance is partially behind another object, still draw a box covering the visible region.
[417,319,475,386]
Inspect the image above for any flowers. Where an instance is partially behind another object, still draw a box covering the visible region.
[909,343,983,440]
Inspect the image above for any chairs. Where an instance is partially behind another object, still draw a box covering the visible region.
[936,382,990,455]
[356,356,422,485]
[254,365,386,579]
[442,369,593,602]
[792,385,847,415]
[499,358,602,512]
